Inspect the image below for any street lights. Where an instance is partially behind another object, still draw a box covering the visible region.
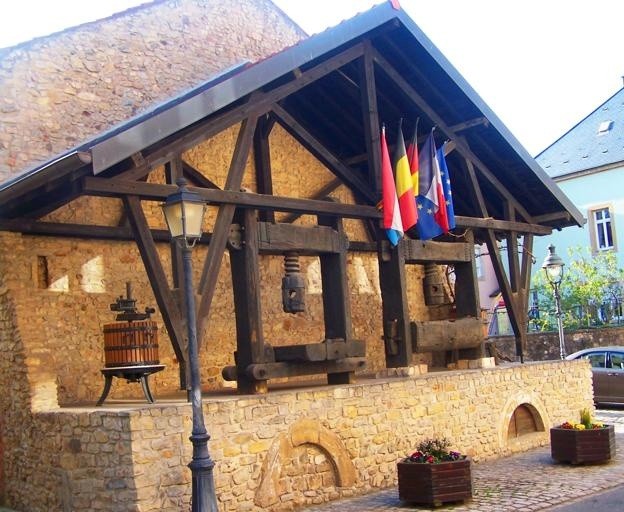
[157,175,219,512]
[542,243,566,361]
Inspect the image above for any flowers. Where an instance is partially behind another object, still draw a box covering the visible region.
[403,436,467,463]
[560,404,606,430]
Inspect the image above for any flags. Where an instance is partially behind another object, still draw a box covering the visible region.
[378,116,456,247]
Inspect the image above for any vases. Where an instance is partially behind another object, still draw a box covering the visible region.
[397,458,472,506]
[550,424,616,465]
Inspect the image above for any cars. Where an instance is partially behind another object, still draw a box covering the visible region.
[566,346,624,409]
